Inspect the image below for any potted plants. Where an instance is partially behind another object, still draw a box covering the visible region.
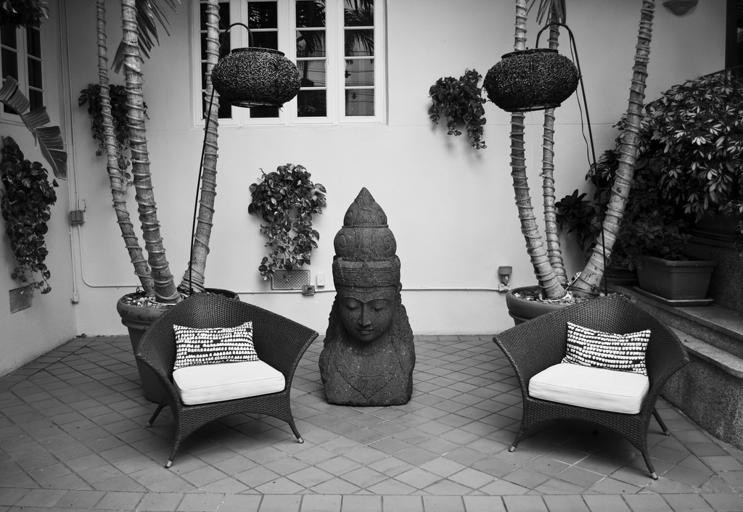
[624,208,715,310]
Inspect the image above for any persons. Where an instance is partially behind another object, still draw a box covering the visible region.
[320,287,414,406]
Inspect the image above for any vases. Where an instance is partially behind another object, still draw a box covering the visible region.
[505,287,630,330]
[117,293,232,406]
[247,163,330,281]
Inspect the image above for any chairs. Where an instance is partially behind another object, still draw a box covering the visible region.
[133,291,320,470]
[492,295,690,481]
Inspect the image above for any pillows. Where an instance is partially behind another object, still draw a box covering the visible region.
[169,320,260,371]
[561,321,652,377]
